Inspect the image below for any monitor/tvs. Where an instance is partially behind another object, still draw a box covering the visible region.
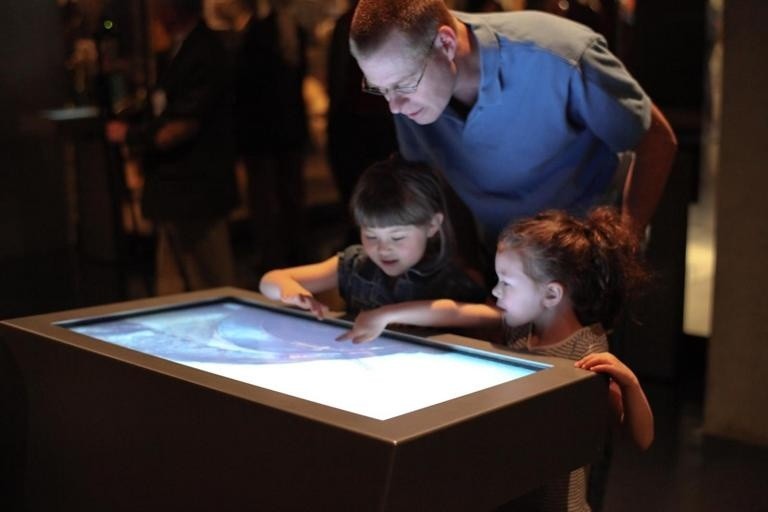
[69,302,539,420]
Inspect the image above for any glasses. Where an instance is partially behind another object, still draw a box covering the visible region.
[362,34,438,96]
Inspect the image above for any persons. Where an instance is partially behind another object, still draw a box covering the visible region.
[259,158,489,339]
[67,0,404,319]
[348,0,677,512]
[525,0,716,298]
[337,211,654,512]
